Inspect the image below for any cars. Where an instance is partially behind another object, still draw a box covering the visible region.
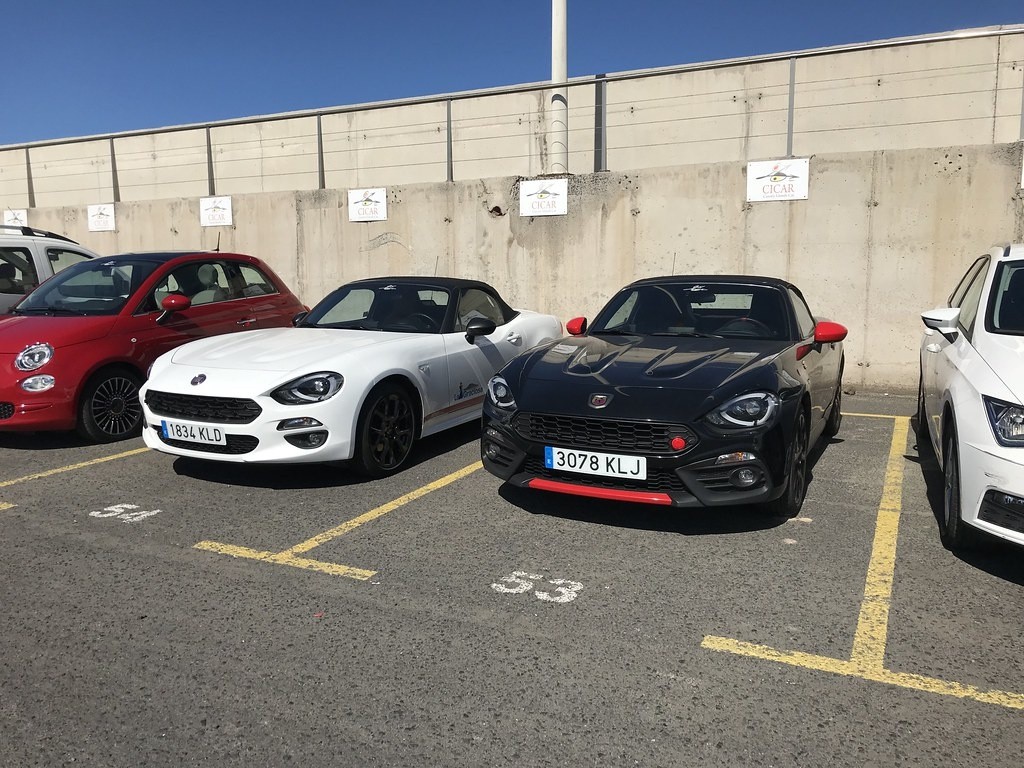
[1,252,312,441]
[0,225,131,313]
[916,243,1024,573]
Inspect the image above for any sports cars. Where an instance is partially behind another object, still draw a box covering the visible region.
[137,274,563,475]
[482,275,846,515]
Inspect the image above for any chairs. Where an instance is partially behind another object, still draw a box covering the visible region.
[639,296,780,339]
[151,263,220,312]
[373,300,446,332]
[0,262,23,294]
